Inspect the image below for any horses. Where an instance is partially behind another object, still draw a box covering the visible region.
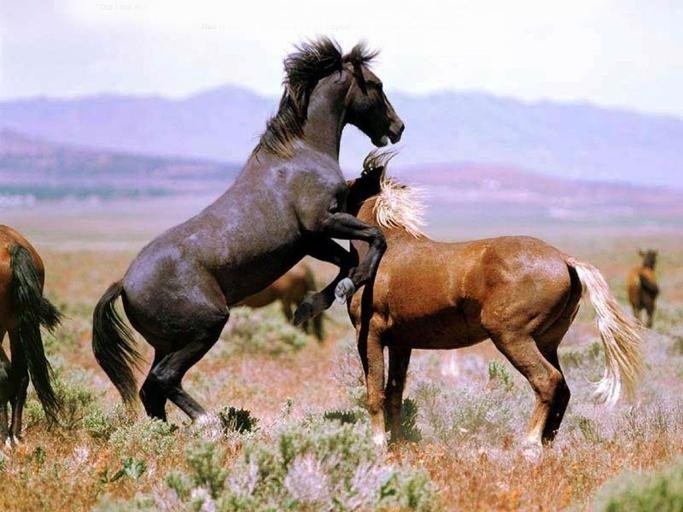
[238,142,661,460]
[90,30,406,427]
[0,224,75,444]
